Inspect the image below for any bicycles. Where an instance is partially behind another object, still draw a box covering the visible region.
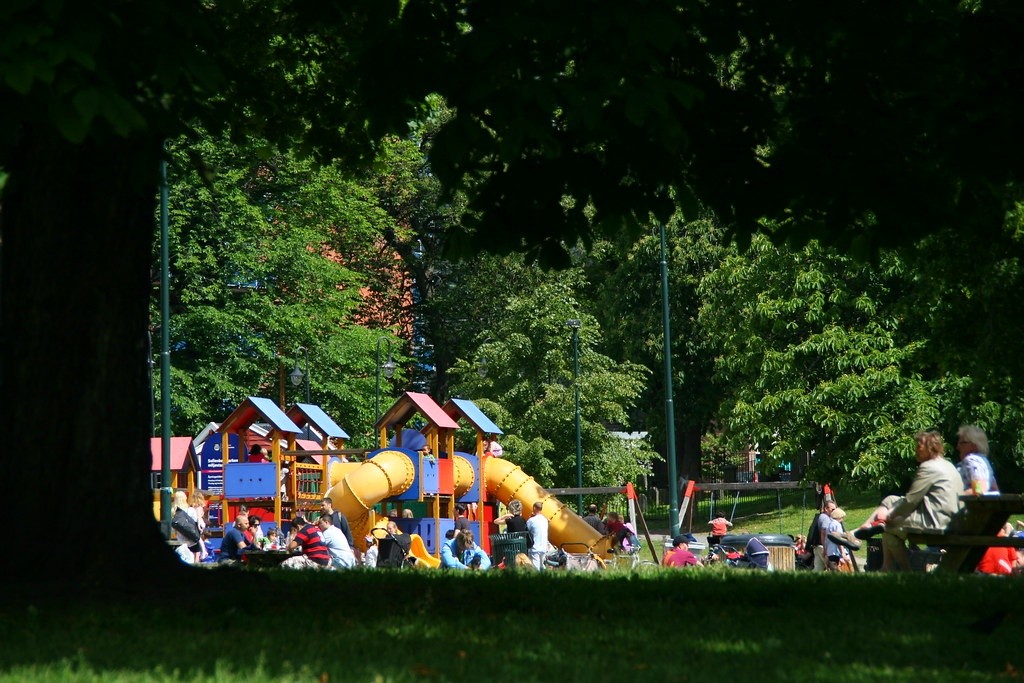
[600,545,660,571]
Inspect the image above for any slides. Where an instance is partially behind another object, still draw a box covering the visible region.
[324,451,441,569]
[484,456,615,563]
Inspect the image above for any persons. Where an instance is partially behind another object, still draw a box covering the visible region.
[386,522,397,534]
[816,482,833,510]
[926,426,998,571]
[494,500,548,571]
[977,523,1024,576]
[662,535,704,567]
[249,443,301,501]
[605,512,642,554]
[472,439,503,458]
[281,498,379,570]
[390,509,413,518]
[585,506,605,535]
[753,470,758,482]
[438,530,492,570]
[423,444,434,459]
[795,534,806,555]
[174,491,208,563]
[708,511,733,549]
[445,505,470,540]
[827,433,963,569]
[219,504,277,566]
[814,501,849,573]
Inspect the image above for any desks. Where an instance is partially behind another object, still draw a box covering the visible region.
[236,547,303,572]
[901,492,1024,577]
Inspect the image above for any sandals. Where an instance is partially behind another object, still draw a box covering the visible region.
[828,531,861,551]
[853,519,888,540]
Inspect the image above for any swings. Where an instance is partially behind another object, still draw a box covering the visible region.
[776,488,806,554]
[706,490,740,545]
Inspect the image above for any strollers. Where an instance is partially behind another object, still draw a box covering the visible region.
[708,537,770,571]
[370,527,417,569]
[543,542,607,574]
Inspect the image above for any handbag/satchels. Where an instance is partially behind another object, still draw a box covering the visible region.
[172,507,200,543]
[526,527,534,548]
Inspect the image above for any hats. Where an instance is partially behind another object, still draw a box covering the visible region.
[673,536,695,546]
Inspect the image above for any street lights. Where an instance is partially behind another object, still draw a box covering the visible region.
[374,336,398,452]
[652,184,680,540]
[289,347,310,440]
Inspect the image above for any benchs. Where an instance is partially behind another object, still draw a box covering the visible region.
[903,526,1024,547]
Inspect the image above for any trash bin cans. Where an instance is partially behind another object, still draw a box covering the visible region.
[489,531,528,568]
[864,538,883,572]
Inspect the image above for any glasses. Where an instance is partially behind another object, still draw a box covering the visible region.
[253,523,260,527]
